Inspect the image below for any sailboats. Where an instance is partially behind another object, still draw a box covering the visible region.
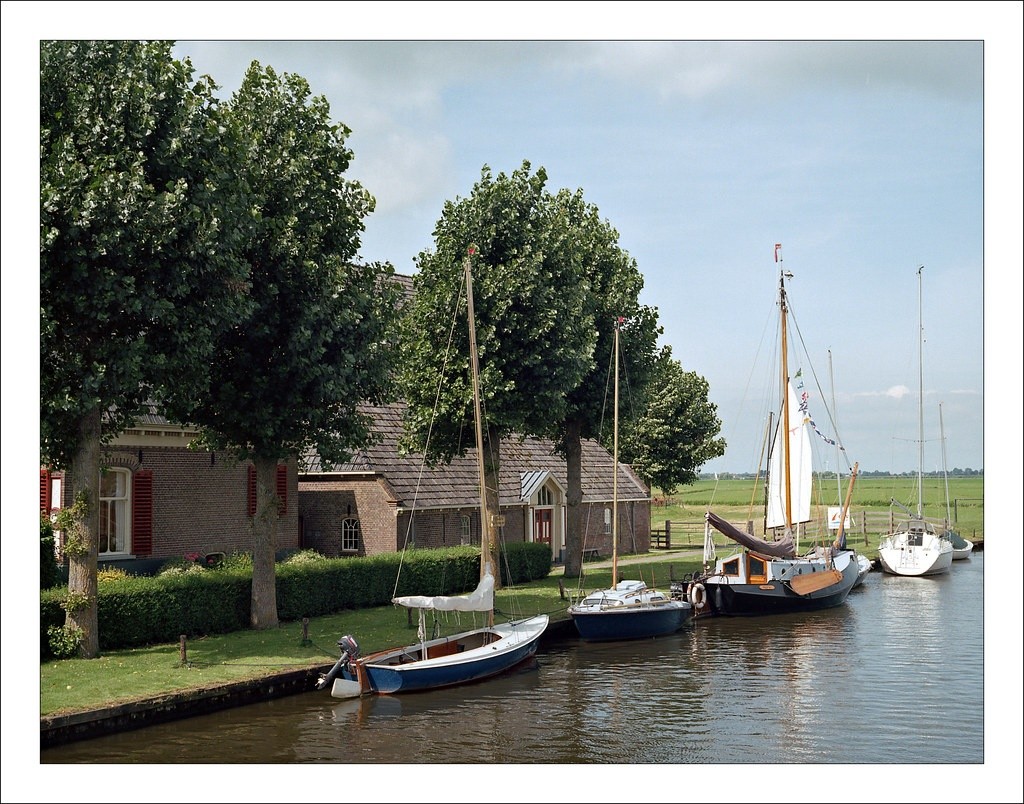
[315,257,550,698]
[681,269,859,617]
[828,350,872,588]
[939,404,974,559]
[877,272,954,576]
[567,328,694,643]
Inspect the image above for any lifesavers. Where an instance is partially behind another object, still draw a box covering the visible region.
[692,583,707,607]
